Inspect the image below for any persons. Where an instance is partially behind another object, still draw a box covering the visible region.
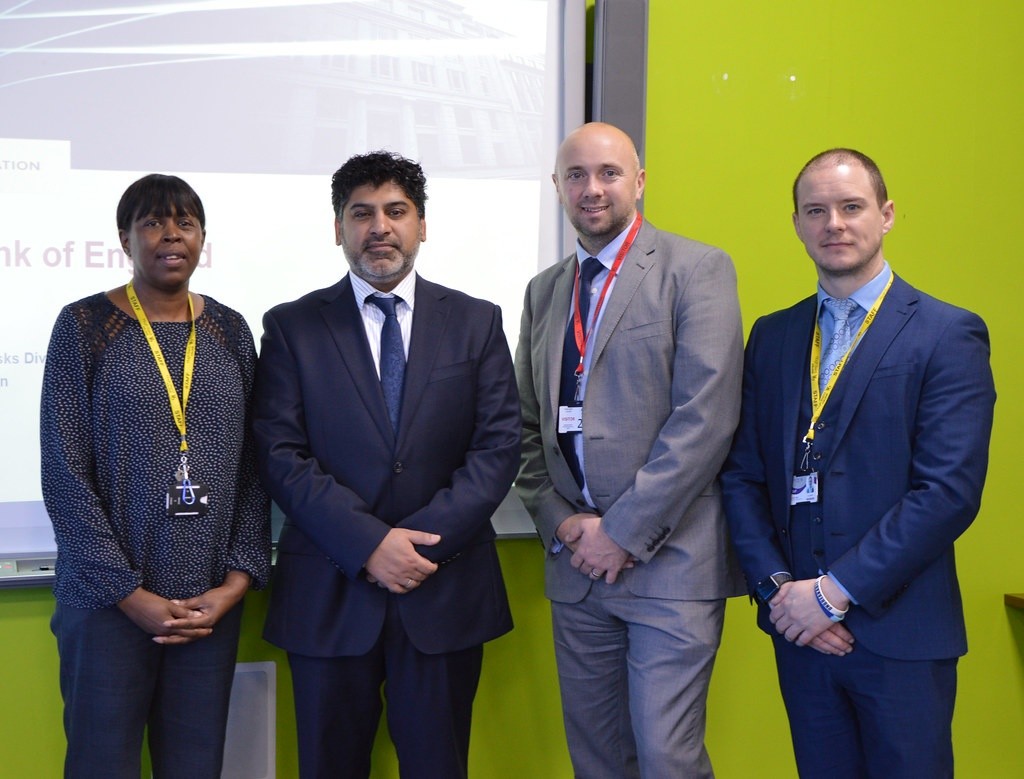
[514,121,744,779]
[717,149,998,779]
[248,151,525,779]
[39,175,273,779]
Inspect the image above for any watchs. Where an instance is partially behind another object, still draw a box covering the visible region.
[752,573,793,606]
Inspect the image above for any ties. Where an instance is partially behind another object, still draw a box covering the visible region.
[557,257,604,490]
[818,298,859,398]
[365,294,407,442]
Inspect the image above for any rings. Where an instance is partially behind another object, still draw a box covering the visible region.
[592,571,601,577]
[402,579,412,588]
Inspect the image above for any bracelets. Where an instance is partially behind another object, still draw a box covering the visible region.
[814,574,850,622]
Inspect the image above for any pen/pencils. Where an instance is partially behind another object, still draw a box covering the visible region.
[39,565,55,570]
